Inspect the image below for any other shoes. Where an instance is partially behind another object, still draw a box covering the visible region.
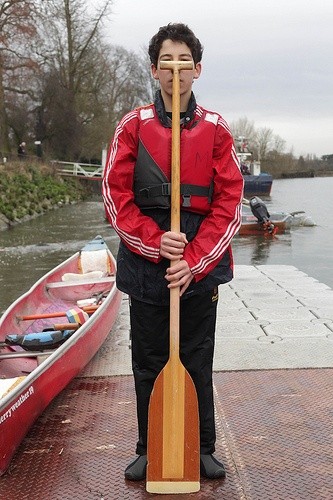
[124,454,146,481]
[200,452,225,478]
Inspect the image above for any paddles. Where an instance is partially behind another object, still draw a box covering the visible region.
[145,50,199,494]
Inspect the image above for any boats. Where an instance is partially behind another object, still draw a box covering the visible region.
[0,234,122,478]
[236,196,306,240]
[235,136,273,197]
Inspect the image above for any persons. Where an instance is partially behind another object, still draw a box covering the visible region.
[17,142,27,159]
[102,24,245,481]
